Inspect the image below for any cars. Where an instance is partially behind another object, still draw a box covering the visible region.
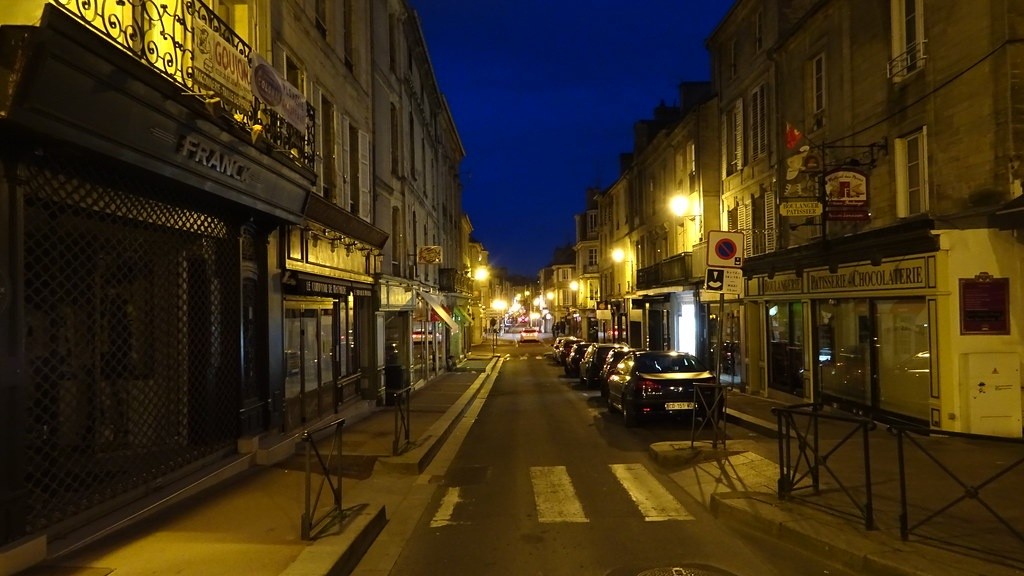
[608,325,626,340]
[712,341,739,372]
[551,336,577,361]
[412,329,441,343]
[609,350,724,427]
[564,343,592,378]
[341,329,354,348]
[600,348,648,402]
[520,328,539,343]
[579,342,632,388]
[881,350,930,413]
[817,342,864,403]
[554,338,585,366]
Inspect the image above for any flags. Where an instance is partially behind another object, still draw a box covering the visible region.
[786,125,802,151]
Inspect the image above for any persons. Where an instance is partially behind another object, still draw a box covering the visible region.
[551,322,559,337]
[590,328,597,342]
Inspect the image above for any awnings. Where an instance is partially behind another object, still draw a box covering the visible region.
[416,290,458,334]
[453,306,474,326]
